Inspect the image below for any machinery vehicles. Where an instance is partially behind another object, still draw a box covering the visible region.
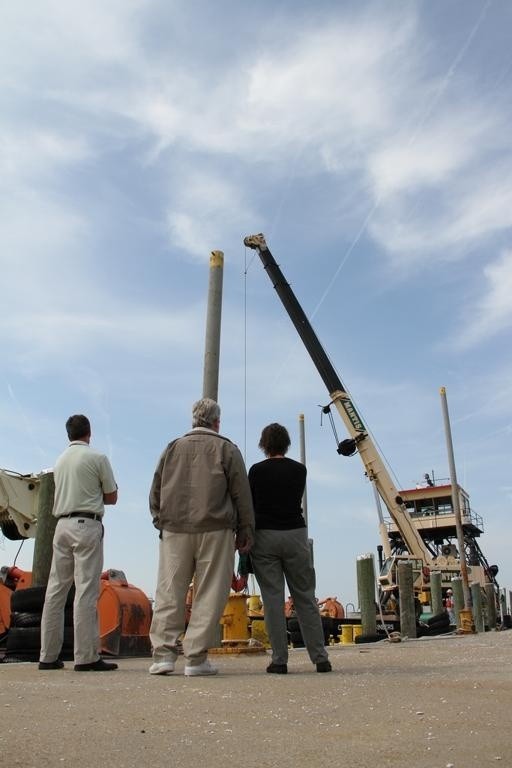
[244,231,491,625]
[0,469,153,663]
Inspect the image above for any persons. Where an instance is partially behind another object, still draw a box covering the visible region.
[240,423,333,673]
[39,415,118,672]
[149,396,258,677]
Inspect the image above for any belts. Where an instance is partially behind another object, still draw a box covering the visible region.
[60,512,102,521]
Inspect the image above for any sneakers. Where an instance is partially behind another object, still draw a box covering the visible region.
[316,660,331,672]
[148,661,175,674]
[184,660,218,676]
[266,663,288,674]
[39,659,64,670]
[74,658,118,671]
